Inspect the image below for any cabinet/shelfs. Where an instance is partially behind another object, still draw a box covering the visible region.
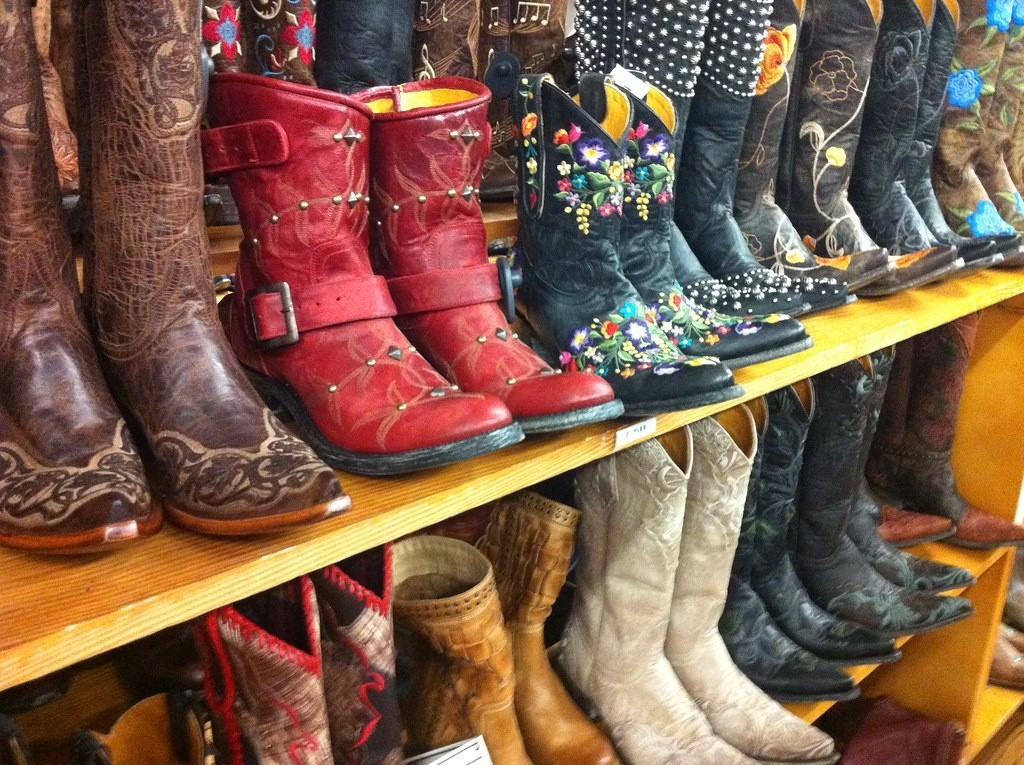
[0,201,1024,765]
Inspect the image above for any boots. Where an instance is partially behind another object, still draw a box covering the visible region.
[0,0,1024,765]
